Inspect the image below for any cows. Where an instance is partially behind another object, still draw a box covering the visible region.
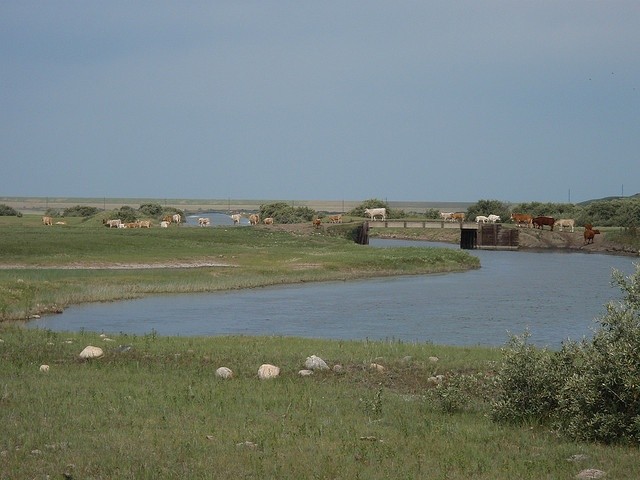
[172,214,182,224]
[139,220,152,228]
[510,212,534,228]
[449,212,465,223]
[584,223,592,230]
[553,218,575,233]
[262,217,274,225]
[127,223,139,228]
[327,215,342,224]
[107,218,122,228]
[439,211,455,221]
[531,216,557,232]
[231,213,242,225]
[312,218,321,229]
[583,229,601,245]
[364,207,387,221]
[41,216,53,225]
[160,216,172,228]
[198,217,210,227]
[248,214,259,225]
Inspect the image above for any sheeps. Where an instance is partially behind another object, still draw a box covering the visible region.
[488,214,501,223]
[475,215,489,224]
